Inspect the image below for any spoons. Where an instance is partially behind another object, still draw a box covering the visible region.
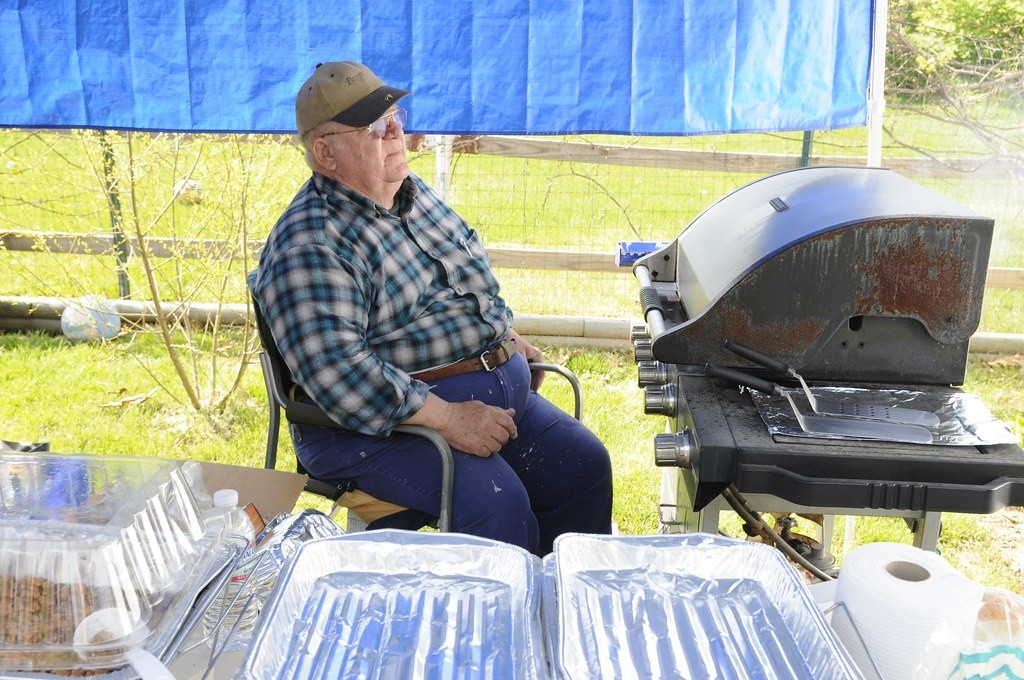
[72,609,178,680]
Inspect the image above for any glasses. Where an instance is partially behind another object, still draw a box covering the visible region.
[318,108,408,140]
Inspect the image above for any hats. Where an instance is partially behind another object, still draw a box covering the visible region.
[295,61,412,135]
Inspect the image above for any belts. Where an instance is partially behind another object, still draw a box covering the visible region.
[409,337,517,382]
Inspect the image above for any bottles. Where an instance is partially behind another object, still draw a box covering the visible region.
[197,489,257,651]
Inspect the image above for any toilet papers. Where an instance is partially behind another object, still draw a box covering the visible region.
[831,541,986,680]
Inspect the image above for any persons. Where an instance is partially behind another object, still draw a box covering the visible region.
[252,54,613,555]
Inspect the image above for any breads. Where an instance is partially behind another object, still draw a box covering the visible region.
[976,597,1023,642]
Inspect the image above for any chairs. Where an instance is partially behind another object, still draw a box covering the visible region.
[251,294,582,533]
